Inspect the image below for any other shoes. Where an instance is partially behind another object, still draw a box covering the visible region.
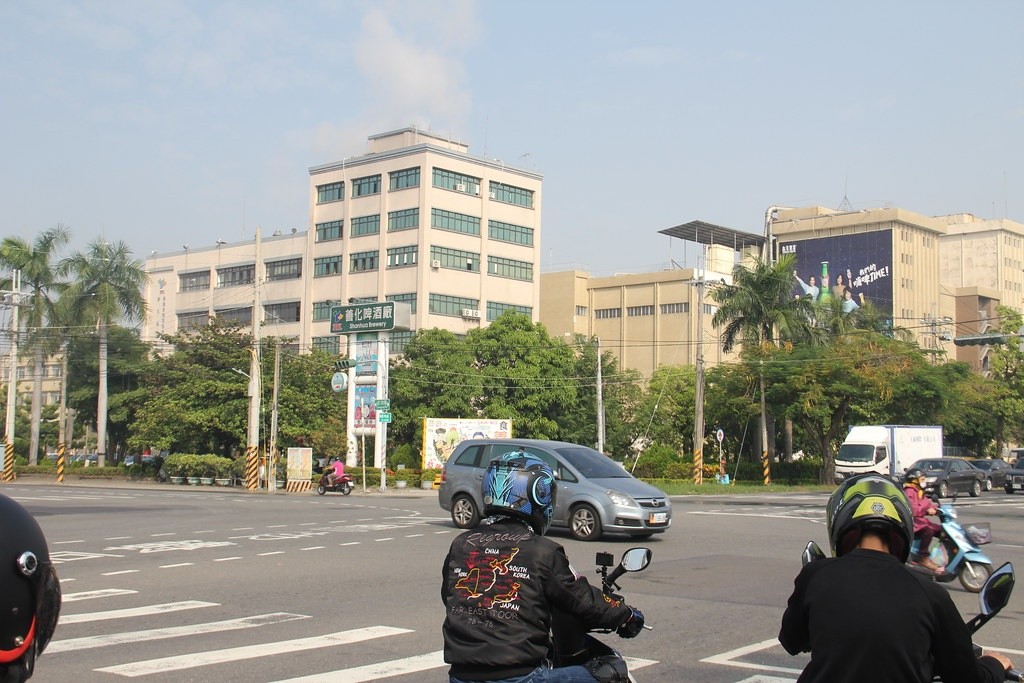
[329,484,334,487]
[913,554,937,570]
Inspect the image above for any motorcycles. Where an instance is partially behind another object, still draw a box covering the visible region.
[317,469,355,496]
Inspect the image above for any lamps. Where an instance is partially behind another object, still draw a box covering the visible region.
[216,238,221,245]
[325,298,375,305]
[152,249,158,255]
[183,244,190,250]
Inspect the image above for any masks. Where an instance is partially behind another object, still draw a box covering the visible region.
[920,483,926,488]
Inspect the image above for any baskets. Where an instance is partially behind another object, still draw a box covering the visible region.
[962,522,991,544]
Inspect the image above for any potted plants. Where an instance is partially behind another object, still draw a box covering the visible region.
[420,471,436,490]
[162,450,286,488]
[394,469,409,488]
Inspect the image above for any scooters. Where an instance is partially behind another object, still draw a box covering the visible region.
[800,540,1024,683]
[545,547,653,683]
[902,489,995,593]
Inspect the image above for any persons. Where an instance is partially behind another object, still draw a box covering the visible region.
[792,270,861,315]
[0,493,62,683]
[779,472,1014,683]
[902,468,943,573]
[326,456,344,487]
[440,452,643,683]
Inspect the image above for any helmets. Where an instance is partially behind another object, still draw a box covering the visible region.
[826,471,915,564]
[906,468,926,480]
[481,451,557,536]
[0,494,62,683]
[335,456,340,461]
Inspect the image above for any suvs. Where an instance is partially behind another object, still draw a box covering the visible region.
[438,438,672,541]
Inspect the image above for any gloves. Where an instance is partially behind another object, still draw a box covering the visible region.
[616,605,644,638]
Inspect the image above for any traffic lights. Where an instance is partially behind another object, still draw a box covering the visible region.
[333,359,357,370]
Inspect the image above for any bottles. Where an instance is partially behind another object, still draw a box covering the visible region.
[816,262,835,303]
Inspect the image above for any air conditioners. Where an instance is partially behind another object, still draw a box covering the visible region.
[489,191,495,199]
[461,309,481,318]
[431,259,440,268]
[456,184,465,191]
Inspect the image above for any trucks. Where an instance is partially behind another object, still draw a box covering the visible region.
[834,424,944,485]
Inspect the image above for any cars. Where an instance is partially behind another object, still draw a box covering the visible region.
[45,451,155,467]
[898,455,1024,498]
